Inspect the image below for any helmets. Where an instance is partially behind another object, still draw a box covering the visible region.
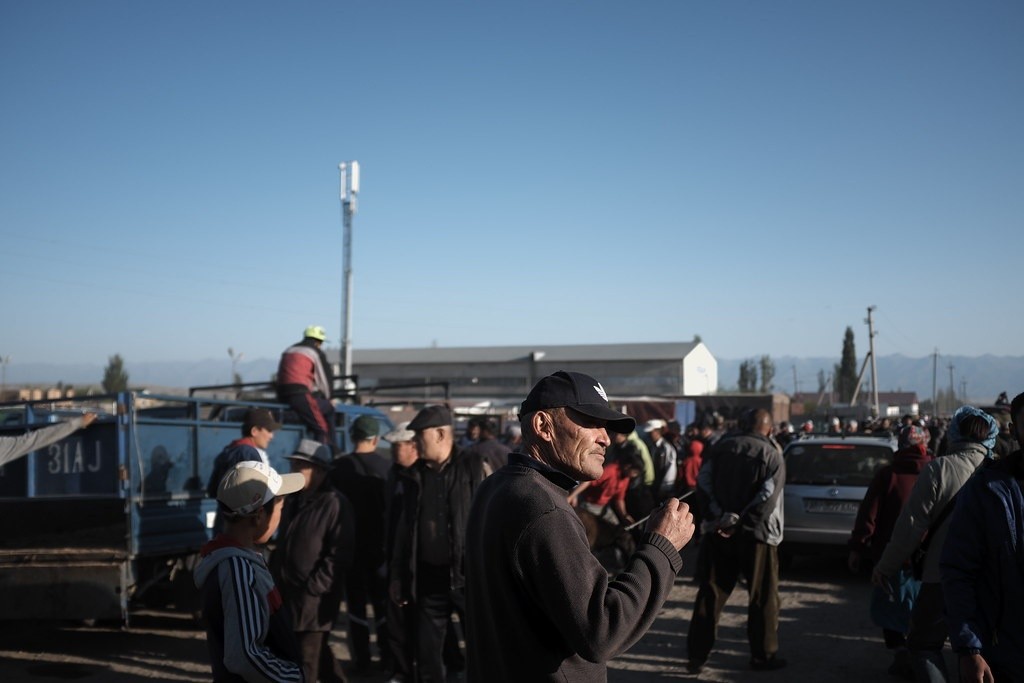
[304,325,326,341]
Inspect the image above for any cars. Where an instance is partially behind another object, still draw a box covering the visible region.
[780,432,898,539]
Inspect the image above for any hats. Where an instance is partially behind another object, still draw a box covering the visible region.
[898,425,930,452]
[381,421,416,443]
[349,414,379,441]
[405,405,452,430]
[215,462,306,518]
[643,419,663,432]
[517,370,635,434]
[242,405,282,431]
[281,439,336,470]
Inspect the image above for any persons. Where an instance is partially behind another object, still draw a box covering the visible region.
[463,369,695,683]
[389,405,493,683]
[277,326,338,444]
[846,424,936,650]
[332,415,514,683]
[205,408,283,540]
[0,412,98,468]
[280,439,355,683]
[937,392,1024,683]
[685,408,789,674]
[871,405,999,683]
[991,412,1021,456]
[193,460,306,683]
[564,417,948,570]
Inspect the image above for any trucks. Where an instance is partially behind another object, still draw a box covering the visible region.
[0,375,403,632]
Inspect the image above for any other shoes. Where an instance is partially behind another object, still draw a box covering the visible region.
[686,663,702,674]
[751,658,788,670]
